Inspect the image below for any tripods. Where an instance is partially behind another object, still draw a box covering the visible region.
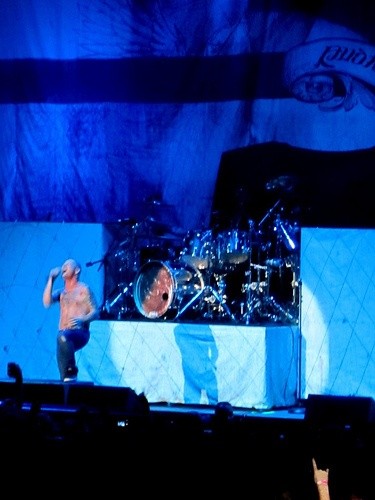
[173,265,299,326]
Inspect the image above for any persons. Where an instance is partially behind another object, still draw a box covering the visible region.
[42,257,100,384]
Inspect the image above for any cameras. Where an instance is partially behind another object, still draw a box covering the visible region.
[115,419,129,429]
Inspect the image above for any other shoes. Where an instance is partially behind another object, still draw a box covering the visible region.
[63,371,79,382]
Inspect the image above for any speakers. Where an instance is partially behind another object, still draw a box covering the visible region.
[305,395,375,422]
[67,384,138,413]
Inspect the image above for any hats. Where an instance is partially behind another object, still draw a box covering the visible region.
[214,402,235,415]
